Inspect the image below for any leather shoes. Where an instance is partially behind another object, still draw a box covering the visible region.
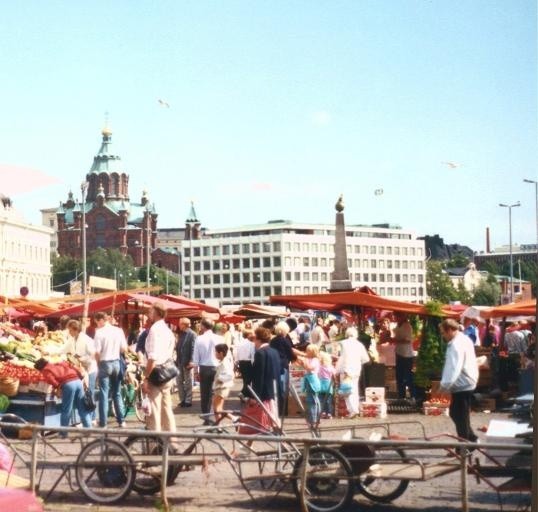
[178,402,193,408]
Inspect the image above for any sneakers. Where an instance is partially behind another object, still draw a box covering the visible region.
[321,411,333,420]
[118,420,127,427]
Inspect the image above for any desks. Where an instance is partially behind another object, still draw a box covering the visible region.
[68,386,103,426]
[0,383,75,441]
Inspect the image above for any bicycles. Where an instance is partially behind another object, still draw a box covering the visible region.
[110,359,152,422]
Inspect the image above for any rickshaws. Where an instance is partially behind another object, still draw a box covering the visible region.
[0,413,227,504]
[425,418,534,511]
[196,408,466,511]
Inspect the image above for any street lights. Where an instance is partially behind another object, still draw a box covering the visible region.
[81,181,90,296]
[145,201,152,295]
[522,179,538,249]
[498,202,519,305]
[512,258,523,297]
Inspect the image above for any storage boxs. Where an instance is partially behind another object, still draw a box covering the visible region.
[420,378,497,416]
[334,385,387,418]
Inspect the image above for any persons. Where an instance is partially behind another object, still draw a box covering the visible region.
[33,304,537,459]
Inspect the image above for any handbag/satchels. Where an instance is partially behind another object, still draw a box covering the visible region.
[82,391,97,412]
[237,396,272,436]
[148,358,180,386]
